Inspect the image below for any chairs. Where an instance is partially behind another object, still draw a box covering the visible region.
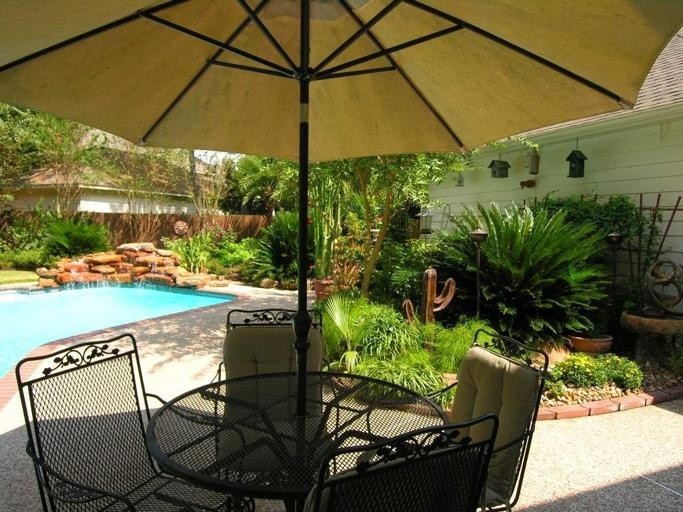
[302,413,499,512]
[424,328,549,512]
[14,332,253,512]
[200,308,335,509]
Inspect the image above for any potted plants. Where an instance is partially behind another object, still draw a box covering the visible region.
[563,207,612,355]
[312,178,334,300]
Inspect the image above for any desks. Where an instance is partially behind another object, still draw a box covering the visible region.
[143,370,452,511]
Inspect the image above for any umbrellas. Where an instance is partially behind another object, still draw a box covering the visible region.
[0,1,683,511]
